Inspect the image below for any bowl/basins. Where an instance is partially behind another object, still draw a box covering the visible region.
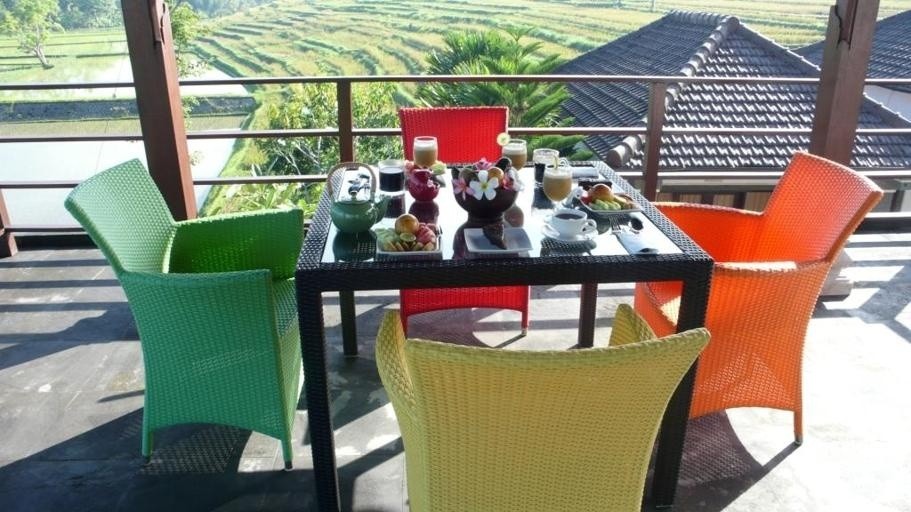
[452,178,519,222]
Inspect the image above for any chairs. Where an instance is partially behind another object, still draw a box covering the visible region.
[375,304,712,512]
[65,157,310,473]
[634,151,883,446]
[400,106,534,339]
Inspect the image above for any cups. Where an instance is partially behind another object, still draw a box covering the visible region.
[501,140,528,190]
[378,159,407,199]
[533,148,560,184]
[543,164,572,221]
[413,136,437,171]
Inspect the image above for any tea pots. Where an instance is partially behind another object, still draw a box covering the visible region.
[327,162,392,233]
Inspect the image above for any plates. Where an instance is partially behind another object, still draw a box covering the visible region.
[580,191,644,214]
[463,228,533,254]
[375,224,444,254]
[540,226,594,243]
